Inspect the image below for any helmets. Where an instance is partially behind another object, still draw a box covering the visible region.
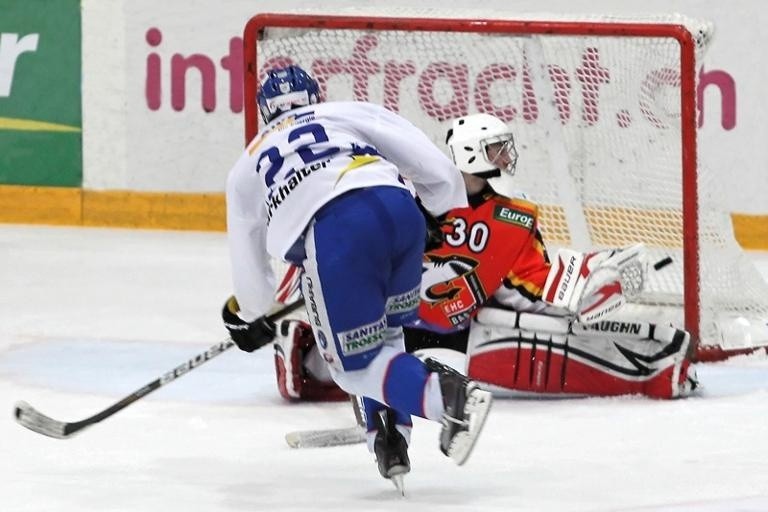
[445,112,518,179]
[256,65,323,124]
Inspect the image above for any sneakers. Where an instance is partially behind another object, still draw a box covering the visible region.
[423,356,479,458]
[372,408,413,478]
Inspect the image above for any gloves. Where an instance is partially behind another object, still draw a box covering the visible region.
[413,192,448,253]
[221,294,276,354]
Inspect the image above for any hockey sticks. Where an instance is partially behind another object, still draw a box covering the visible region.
[287,392,371,449]
[12,297,304,439]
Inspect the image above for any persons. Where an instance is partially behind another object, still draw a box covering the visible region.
[273,114,697,398]
[226,65,493,497]
